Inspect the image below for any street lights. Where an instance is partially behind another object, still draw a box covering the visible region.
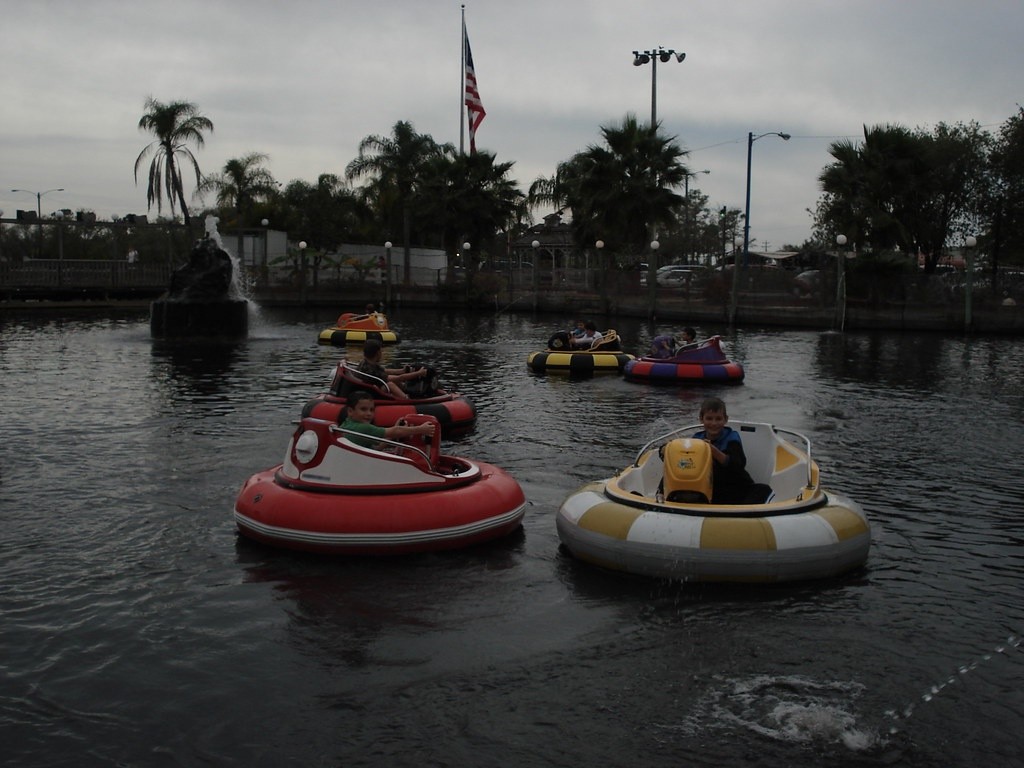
[594,240,606,304]
[837,234,847,283]
[298,241,308,304]
[261,218,268,261]
[731,234,744,305]
[10,189,65,258]
[742,130,792,284]
[385,241,393,302]
[649,240,661,312]
[631,46,687,240]
[462,243,473,296]
[530,240,541,296]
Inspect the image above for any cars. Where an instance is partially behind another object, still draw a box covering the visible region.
[636,262,823,300]
[919,263,1024,308]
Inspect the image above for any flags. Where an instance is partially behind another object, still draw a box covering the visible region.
[464,26,487,157]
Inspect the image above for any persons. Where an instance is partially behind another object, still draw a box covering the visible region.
[376,256,386,284]
[338,390,436,451]
[570,320,602,350]
[128,244,138,280]
[655,396,755,504]
[661,327,698,356]
[354,304,374,320]
[355,339,428,400]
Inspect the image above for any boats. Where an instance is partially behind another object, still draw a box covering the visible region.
[527,328,635,376]
[318,312,402,345]
[555,419,872,589]
[233,415,527,556]
[301,356,477,439]
[623,335,746,386]
